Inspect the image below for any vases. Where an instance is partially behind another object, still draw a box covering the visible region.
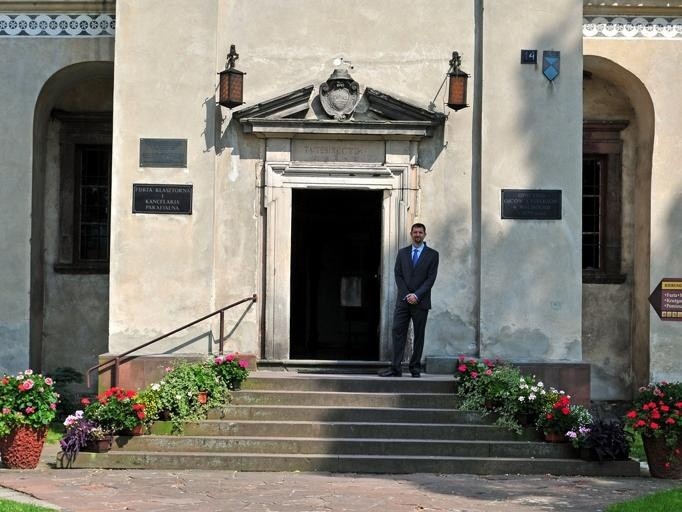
[1,418,51,469]
[638,428,682,480]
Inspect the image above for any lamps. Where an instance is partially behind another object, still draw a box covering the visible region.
[214,41,248,111]
[442,50,473,114]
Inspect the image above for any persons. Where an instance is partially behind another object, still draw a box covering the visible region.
[378,222,440,377]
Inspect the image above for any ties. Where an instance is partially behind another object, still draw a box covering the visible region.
[412,249,419,270]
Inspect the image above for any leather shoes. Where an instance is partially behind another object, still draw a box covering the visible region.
[377,367,403,377]
[411,372,420,377]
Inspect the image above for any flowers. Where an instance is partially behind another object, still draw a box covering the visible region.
[450,348,635,468]
[55,350,252,470]
[620,374,682,471]
[1,367,63,443]
